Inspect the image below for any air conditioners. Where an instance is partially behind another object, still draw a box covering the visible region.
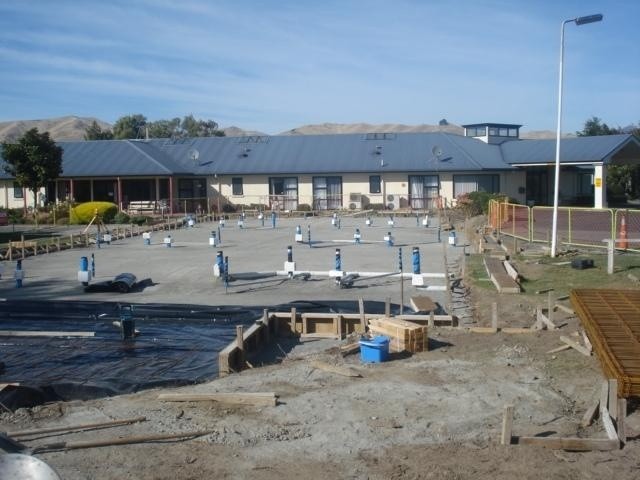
[385,194,400,210]
[348,192,362,211]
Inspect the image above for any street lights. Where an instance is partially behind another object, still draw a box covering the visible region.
[550,13,603,259]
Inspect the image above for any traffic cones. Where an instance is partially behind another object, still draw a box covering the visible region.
[616,214,628,250]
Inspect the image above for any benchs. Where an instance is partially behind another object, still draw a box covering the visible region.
[126,200,171,215]
